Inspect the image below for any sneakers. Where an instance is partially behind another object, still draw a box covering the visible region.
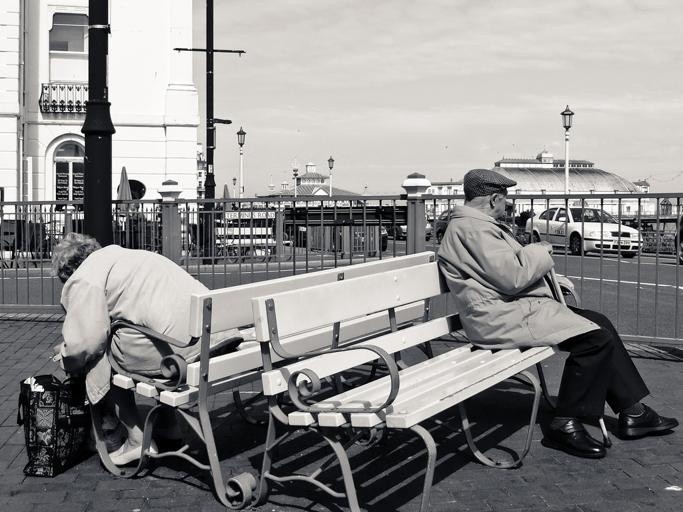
[109,439,159,465]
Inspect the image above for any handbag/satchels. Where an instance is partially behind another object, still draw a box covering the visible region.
[17,374,89,477]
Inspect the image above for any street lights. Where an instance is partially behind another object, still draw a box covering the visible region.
[235,125,247,200]
[327,154,334,195]
[559,104,574,205]
[291,167,298,198]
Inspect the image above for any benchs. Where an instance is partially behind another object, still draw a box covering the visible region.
[239,260,583,512]
[89,251,436,509]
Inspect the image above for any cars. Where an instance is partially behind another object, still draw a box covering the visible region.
[673,213,683,264]
[523,206,643,260]
[280,204,526,257]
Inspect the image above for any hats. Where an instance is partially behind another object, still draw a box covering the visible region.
[464,169,517,198]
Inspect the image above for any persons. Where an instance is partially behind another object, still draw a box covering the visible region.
[437,169,679,459]
[52,231,244,467]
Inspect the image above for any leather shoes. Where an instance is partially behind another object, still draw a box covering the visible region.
[542,419,606,459]
[618,403,679,440]
